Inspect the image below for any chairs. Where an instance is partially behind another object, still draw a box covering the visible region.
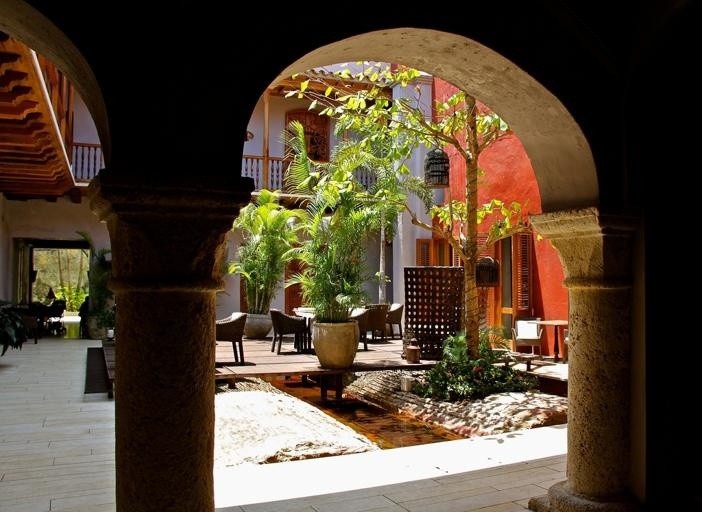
[14,303,37,345]
[269,302,403,354]
[215,311,247,366]
[511,317,544,361]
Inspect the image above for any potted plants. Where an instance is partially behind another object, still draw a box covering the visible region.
[227,189,300,338]
[224,118,436,368]
[74,229,117,340]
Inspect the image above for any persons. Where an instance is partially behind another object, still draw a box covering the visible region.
[78,295,90,339]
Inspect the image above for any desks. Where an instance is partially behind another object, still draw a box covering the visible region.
[528,320,568,362]
